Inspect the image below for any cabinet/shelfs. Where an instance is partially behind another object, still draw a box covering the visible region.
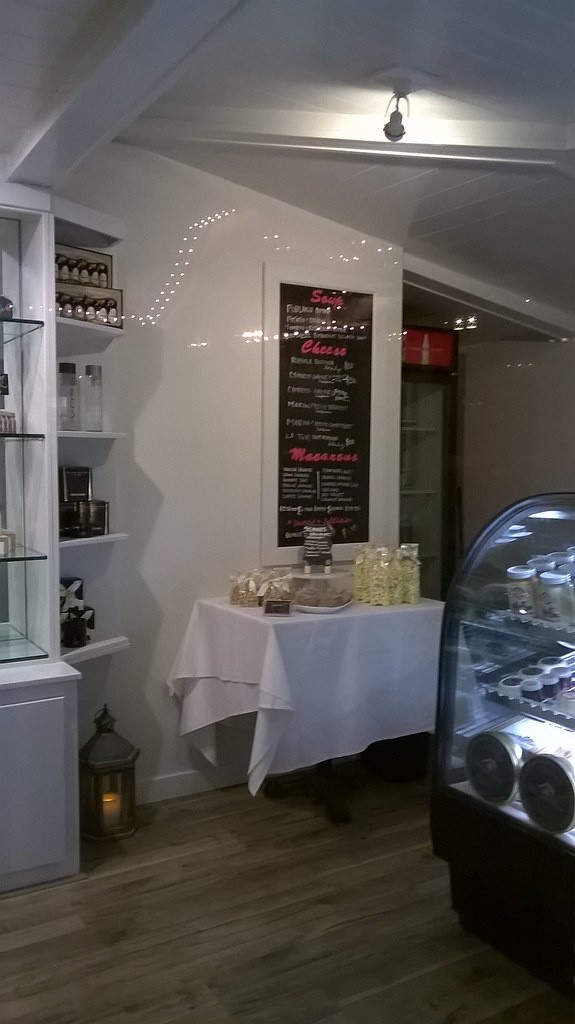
[1,205,131,895]
[399,425,439,561]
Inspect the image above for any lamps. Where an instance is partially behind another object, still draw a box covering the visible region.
[367,63,441,143]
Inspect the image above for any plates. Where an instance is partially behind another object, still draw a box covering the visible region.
[293,599,354,614]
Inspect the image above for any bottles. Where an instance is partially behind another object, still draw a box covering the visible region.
[57,362,81,431]
[84,364,103,432]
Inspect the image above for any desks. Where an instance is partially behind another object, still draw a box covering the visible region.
[166,596,447,826]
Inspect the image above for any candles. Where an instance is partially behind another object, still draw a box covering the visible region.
[101,793,120,826]
